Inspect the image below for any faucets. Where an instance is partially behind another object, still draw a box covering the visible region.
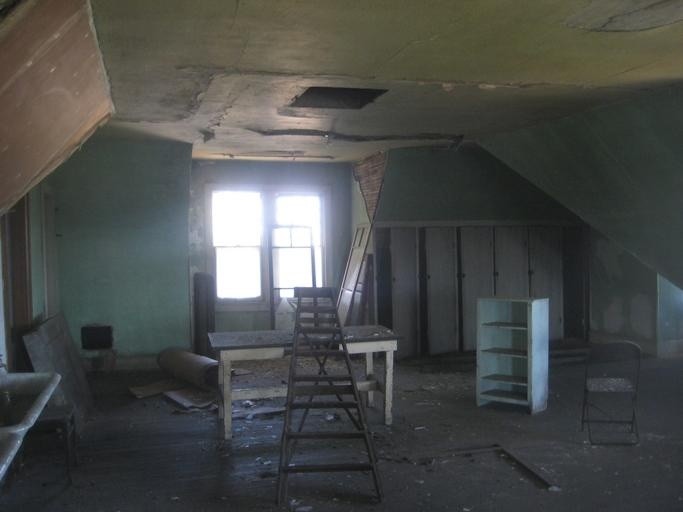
[0,353,9,370]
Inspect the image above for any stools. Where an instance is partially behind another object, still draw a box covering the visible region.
[28,404,75,486]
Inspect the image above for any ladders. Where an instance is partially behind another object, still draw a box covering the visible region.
[275,286,384,512]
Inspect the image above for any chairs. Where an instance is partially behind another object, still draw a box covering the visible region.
[580,340,642,446]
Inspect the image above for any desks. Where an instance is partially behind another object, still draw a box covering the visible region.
[207,324,398,440]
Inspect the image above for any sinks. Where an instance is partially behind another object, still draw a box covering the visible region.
[0,373,59,429]
[0,434,17,470]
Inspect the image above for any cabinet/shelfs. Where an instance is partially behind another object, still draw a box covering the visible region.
[476,297,549,416]
